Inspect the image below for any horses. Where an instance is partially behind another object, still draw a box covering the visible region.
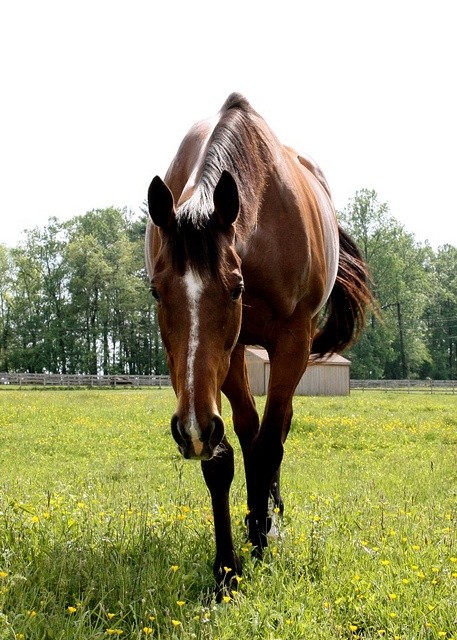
[143,92,389,607]
